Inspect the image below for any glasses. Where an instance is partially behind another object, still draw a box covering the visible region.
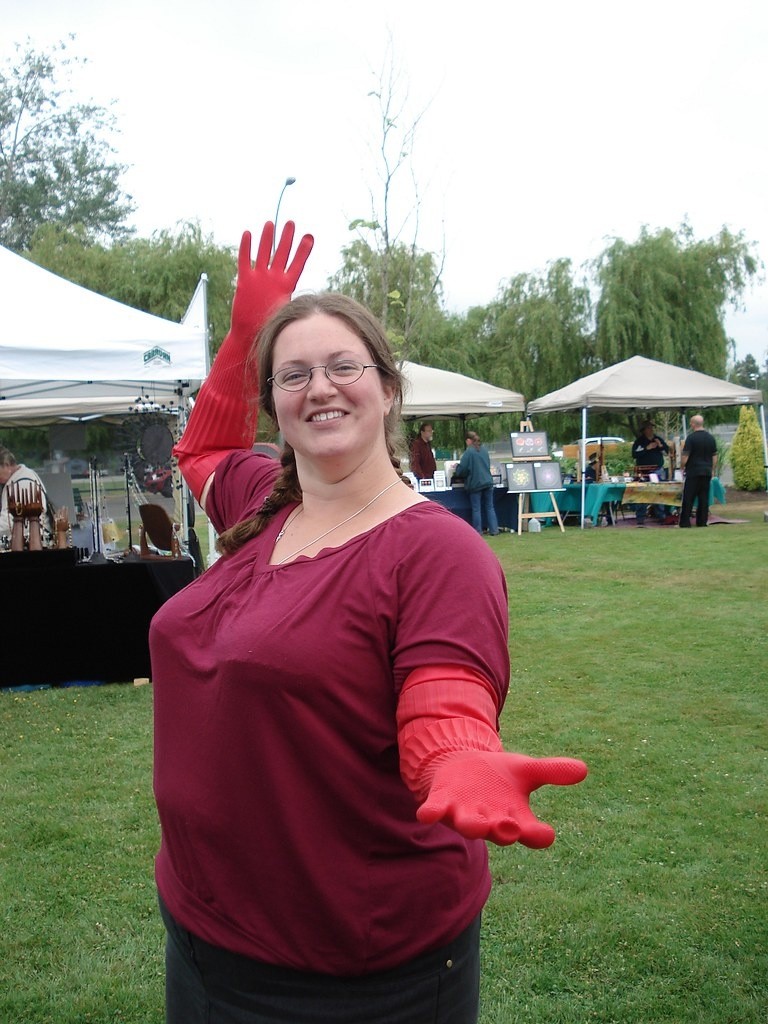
[267,359,378,391]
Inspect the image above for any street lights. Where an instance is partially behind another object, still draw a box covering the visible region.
[271,177,297,255]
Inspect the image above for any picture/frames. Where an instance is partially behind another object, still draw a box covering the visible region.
[532,461,562,489]
[510,430,549,456]
[505,462,536,490]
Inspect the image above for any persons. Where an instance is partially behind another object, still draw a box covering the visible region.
[0,445,56,548]
[150,221,586,1024]
[679,414,718,527]
[453,431,502,536]
[585,452,618,525]
[632,419,669,528]
[410,423,437,482]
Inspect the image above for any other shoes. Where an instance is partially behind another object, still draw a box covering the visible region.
[637,519,644,524]
[675,525,683,528]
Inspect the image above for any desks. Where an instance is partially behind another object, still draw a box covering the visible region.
[418,485,519,532]
[0,547,195,689]
[531,476,728,526]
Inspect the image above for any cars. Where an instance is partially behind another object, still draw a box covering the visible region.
[145,468,174,499]
[569,436,626,445]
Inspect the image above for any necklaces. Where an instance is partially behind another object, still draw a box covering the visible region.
[267,478,402,564]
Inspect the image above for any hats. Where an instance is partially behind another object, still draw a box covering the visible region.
[639,420,656,431]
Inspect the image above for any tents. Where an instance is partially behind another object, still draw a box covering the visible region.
[386,359,526,531]
[523,356,768,532]
[0,241,211,573]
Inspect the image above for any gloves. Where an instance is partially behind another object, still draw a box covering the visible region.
[171,219,314,508]
[395,665,588,849]
